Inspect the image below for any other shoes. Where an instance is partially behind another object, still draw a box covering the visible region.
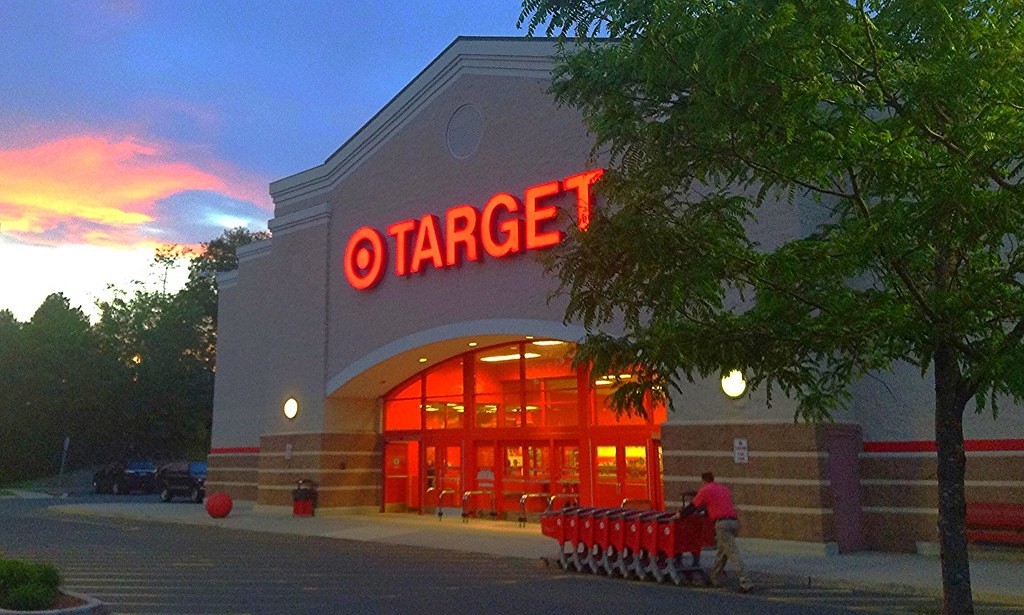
[734,585,754,594]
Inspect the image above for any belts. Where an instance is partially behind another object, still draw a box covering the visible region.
[714,517,737,523]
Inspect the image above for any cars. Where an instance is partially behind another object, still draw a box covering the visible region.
[145,461,206,503]
[92,460,157,496]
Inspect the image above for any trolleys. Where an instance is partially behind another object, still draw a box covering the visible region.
[537,491,714,590]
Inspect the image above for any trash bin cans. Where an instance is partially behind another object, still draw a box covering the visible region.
[292,479,318,517]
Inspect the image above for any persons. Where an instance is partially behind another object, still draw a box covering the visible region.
[675,472,754,592]
